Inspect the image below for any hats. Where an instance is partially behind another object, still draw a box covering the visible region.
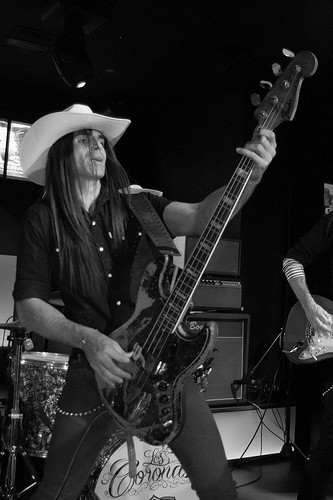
[19,103,126,187]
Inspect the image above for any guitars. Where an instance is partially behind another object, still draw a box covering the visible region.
[278,294,333,364]
[95,48,321,447]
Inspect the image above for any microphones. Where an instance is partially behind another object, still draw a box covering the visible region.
[232,379,255,384]
[12,315,34,350]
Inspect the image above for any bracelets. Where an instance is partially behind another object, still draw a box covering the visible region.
[82,329,98,349]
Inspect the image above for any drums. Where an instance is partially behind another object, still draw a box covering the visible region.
[5,350,71,459]
[83,430,200,500]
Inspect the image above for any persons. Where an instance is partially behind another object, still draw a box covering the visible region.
[12,104,276,500]
[282,210,333,500]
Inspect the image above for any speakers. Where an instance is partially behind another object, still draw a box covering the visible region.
[189,311,250,404]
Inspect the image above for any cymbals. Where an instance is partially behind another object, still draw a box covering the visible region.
[0,319,27,332]
